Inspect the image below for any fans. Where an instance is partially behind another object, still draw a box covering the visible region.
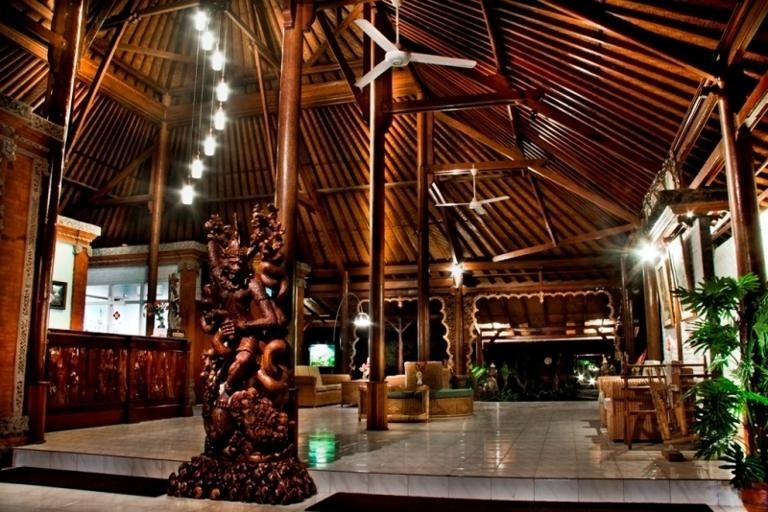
[352,2,479,91]
[434,165,513,217]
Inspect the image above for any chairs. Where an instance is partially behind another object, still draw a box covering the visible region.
[598,358,696,440]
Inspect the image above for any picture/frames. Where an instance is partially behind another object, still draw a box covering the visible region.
[48,279,68,310]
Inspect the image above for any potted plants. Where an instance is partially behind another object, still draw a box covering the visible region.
[667,271,767,512]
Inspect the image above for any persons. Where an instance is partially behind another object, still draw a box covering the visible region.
[192,203,291,394]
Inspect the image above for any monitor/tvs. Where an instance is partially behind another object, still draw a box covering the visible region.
[307,342,338,369]
[306,431,338,468]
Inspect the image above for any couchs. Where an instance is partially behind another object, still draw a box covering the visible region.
[294,363,352,408]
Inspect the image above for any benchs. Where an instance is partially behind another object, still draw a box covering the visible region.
[385,387,475,417]
